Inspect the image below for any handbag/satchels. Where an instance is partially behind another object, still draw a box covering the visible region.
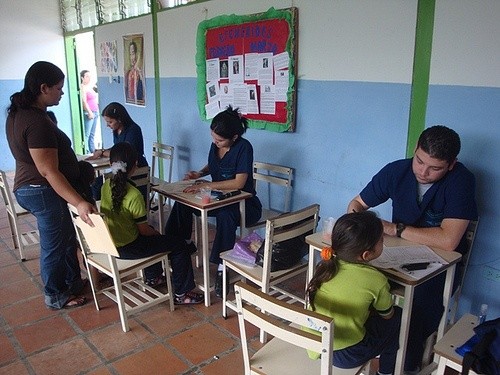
[455,317,500,375]
[255,212,320,271]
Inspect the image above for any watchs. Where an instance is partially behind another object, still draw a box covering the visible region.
[101,149,106,155]
[396,222,408,238]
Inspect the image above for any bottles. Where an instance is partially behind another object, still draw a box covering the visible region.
[477,304,488,325]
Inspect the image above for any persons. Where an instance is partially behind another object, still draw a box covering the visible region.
[100,142,205,306]
[165,105,262,298]
[297,210,420,375]
[5,61,99,312]
[347,125,479,365]
[84,102,151,201]
[79,69,99,155]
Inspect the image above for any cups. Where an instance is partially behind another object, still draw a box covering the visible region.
[322,218,336,243]
[200,186,212,205]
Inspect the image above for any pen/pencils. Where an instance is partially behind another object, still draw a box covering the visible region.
[184,172,204,175]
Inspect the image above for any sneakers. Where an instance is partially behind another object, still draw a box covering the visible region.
[174,291,204,304]
[144,275,167,287]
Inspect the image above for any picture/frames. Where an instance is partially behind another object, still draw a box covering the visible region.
[123,33,145,107]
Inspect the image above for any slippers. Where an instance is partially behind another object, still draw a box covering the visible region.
[47,294,88,311]
[73,276,88,291]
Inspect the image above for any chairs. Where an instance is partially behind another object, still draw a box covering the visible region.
[0,138,500,375]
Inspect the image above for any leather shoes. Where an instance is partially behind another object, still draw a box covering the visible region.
[215,270,229,297]
[187,241,197,255]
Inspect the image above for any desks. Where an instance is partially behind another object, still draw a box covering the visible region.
[75,152,111,176]
[304,231,462,375]
[152,179,252,308]
